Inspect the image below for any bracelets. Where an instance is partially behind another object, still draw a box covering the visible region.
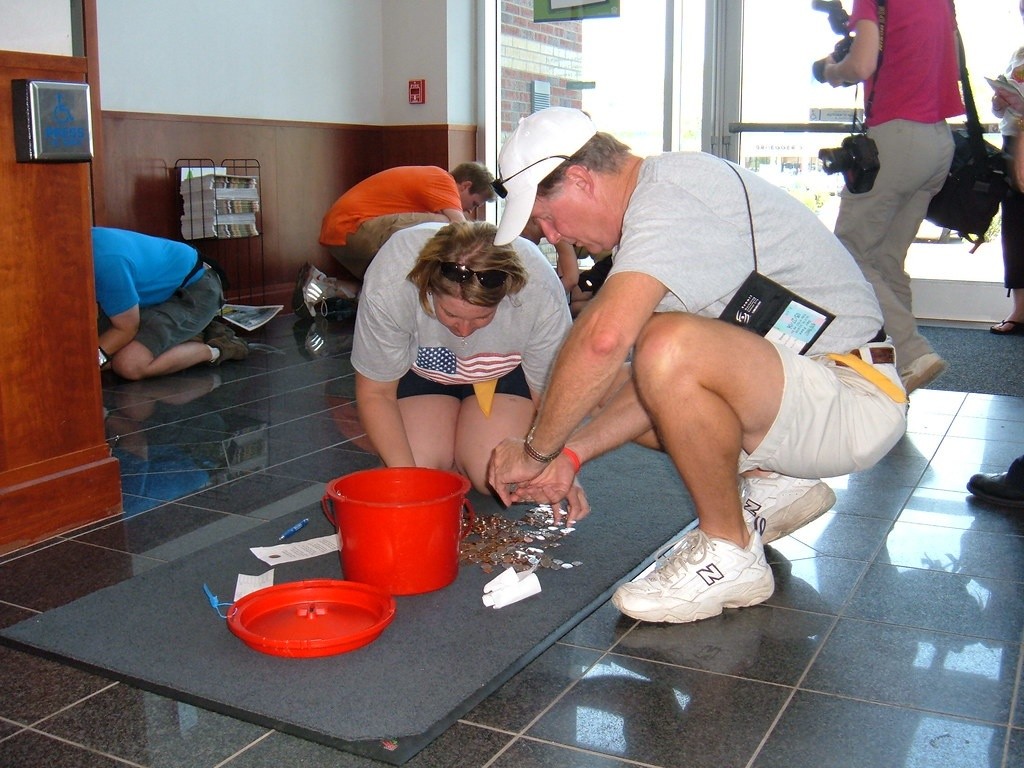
[992,106,1002,111]
[560,446,580,474]
[1017,118,1024,130]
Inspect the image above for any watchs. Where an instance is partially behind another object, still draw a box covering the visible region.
[523,423,566,460]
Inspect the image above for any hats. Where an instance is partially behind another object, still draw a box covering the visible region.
[493,106,598,246]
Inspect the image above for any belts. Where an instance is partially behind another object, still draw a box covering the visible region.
[836,347,895,366]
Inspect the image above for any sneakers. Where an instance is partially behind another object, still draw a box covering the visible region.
[185,332,204,342]
[293,320,326,362]
[741,471,836,544]
[292,262,334,320]
[204,321,251,367]
[611,527,775,624]
[896,351,946,396]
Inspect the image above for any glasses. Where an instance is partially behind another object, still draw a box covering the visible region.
[489,155,572,198]
[437,261,509,289]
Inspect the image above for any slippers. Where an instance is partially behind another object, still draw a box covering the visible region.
[989,319,1024,334]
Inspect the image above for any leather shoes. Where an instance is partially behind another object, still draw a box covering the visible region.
[966,471,1023,506]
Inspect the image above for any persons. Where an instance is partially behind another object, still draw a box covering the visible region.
[519,212,617,316]
[965,0,1024,524]
[484,107,912,627]
[87,223,251,377]
[814,0,966,400]
[294,160,498,321]
[347,218,592,533]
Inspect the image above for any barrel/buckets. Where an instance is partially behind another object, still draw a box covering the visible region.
[321,466,473,598]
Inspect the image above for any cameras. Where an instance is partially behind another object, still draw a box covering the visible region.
[577,253,613,293]
[818,134,881,194]
[812,0,860,87]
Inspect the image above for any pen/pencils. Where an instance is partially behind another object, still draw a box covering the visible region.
[273,517,309,546]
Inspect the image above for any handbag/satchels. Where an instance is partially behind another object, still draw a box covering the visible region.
[924,128,1008,254]
